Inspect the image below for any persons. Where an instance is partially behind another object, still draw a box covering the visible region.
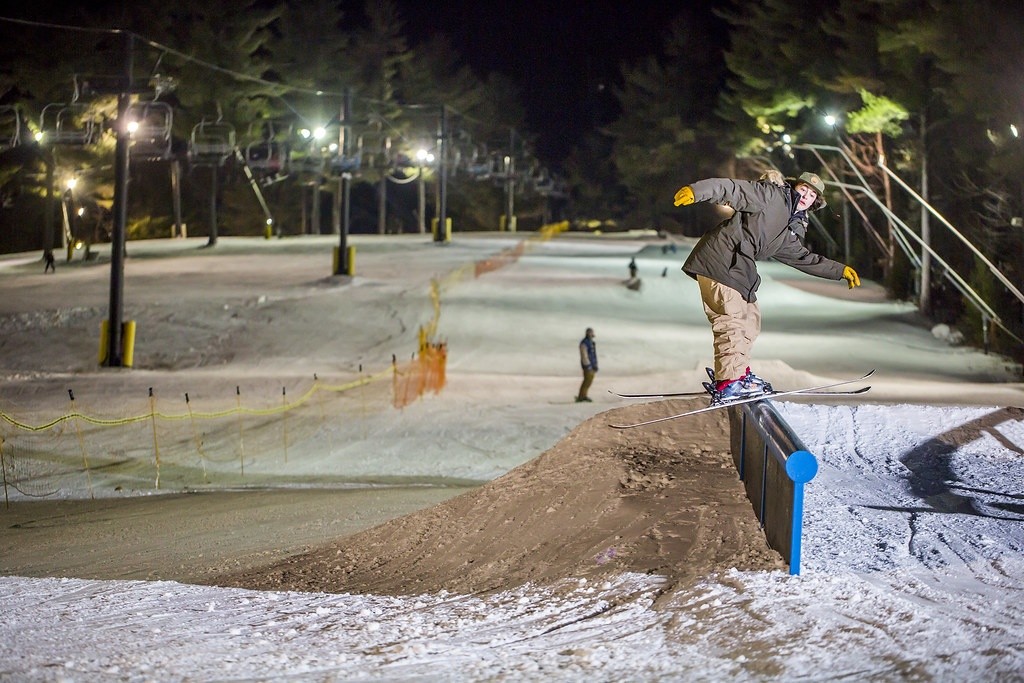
[44,249,55,273]
[674,171,861,403]
[629,258,636,277]
[576,328,599,402]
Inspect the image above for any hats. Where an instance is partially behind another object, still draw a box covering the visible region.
[798,172,825,196]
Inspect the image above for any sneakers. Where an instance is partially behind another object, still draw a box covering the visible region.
[741,373,768,387]
[712,378,765,400]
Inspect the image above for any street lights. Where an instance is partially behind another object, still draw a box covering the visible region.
[826,111,851,267]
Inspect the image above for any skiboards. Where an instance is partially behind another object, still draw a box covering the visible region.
[547,393,623,405]
[608,368,876,429]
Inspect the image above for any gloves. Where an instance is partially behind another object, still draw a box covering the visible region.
[842,266,861,289]
[674,185,696,206]
